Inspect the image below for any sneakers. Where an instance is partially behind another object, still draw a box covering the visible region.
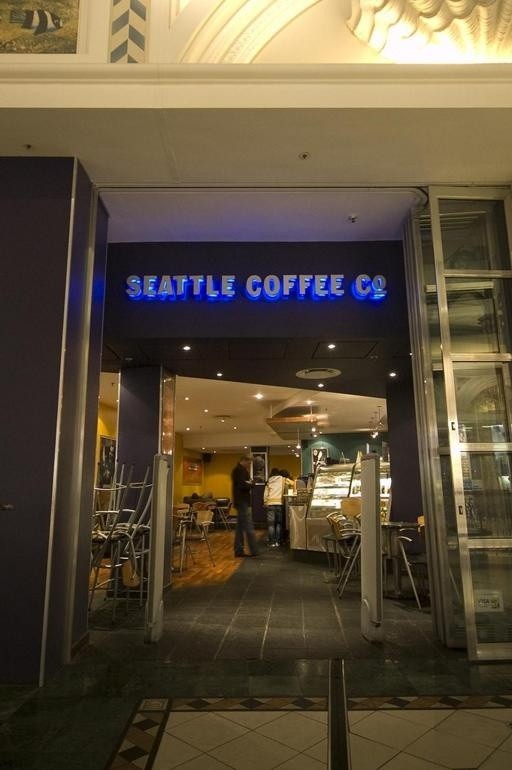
[234,543,278,557]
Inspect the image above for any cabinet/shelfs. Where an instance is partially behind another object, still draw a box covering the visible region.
[305,462,392,554]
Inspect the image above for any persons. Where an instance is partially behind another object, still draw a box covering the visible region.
[320,449,327,461]
[253,455,266,483]
[230,454,265,559]
[100,442,115,483]
[313,449,318,465]
[263,466,294,549]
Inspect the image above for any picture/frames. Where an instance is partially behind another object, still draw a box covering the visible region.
[310,447,329,473]
[250,452,268,486]
[97,434,118,489]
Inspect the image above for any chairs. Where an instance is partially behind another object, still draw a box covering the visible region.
[94,465,240,641]
[323,511,428,612]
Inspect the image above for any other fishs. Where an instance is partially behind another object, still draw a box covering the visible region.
[10,7,63,38]
